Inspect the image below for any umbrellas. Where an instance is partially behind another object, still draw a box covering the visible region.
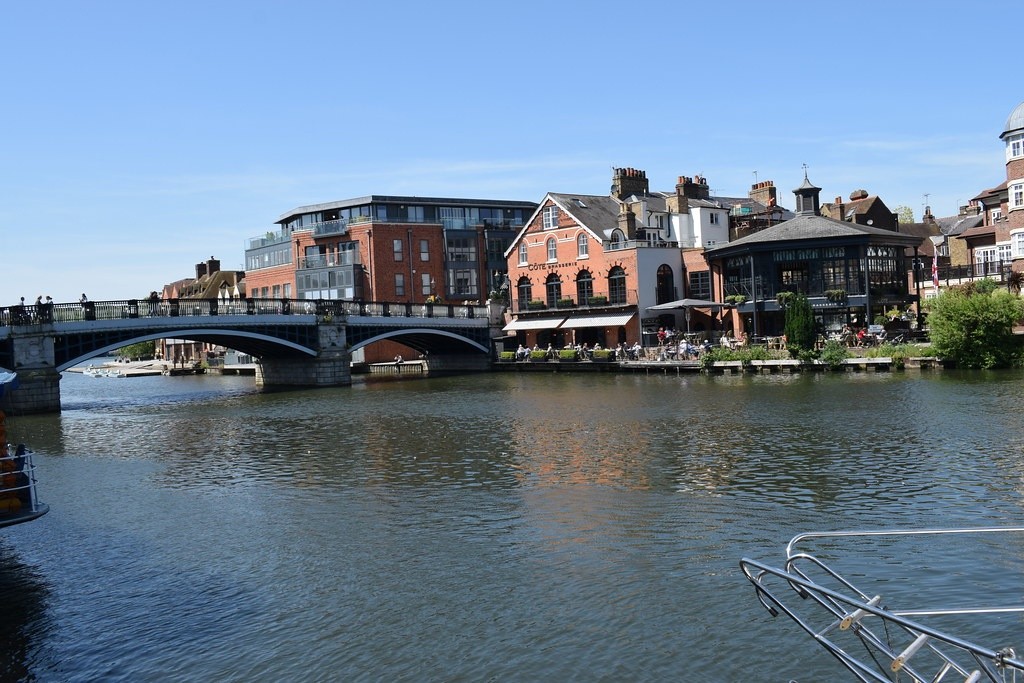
[645,298,730,333]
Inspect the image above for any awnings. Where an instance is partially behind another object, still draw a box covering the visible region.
[502,316,567,330]
[559,312,635,328]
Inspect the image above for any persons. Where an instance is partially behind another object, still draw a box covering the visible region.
[616,341,641,360]
[181,356,184,368]
[547,343,552,350]
[720,332,746,350]
[35,296,54,321]
[516,344,531,357]
[149,291,159,300]
[569,342,601,360]
[394,353,403,363]
[657,326,696,360]
[841,326,886,346]
[704,339,712,353]
[533,343,539,351]
[19,297,24,305]
[174,356,178,368]
[79,293,87,318]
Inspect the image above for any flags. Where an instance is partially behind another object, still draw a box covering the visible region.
[932,250,939,286]
